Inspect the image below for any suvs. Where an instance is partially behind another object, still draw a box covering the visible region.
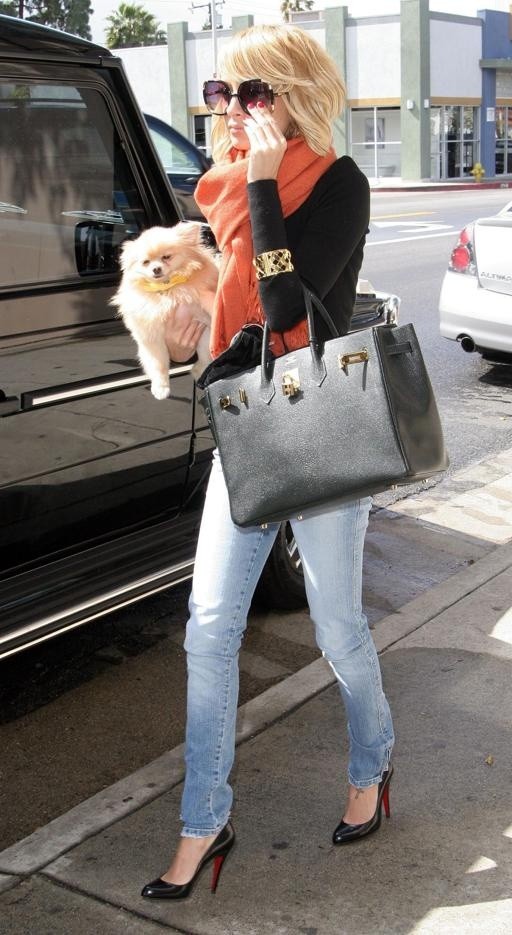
[1,14,404,670]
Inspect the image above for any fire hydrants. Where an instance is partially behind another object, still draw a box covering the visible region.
[470,161,486,185]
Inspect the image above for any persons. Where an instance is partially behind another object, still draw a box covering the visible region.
[138,24,398,904]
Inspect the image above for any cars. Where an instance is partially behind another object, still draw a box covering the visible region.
[0,99,210,229]
[440,200,512,375]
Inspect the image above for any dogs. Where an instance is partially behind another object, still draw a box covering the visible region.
[105,218,226,400]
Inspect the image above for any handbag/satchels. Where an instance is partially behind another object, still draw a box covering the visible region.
[205,284,444,531]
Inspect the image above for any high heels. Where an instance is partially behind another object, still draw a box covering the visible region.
[333,764,394,843]
[140,822,238,901]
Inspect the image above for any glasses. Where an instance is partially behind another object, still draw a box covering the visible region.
[201,77,278,117]
